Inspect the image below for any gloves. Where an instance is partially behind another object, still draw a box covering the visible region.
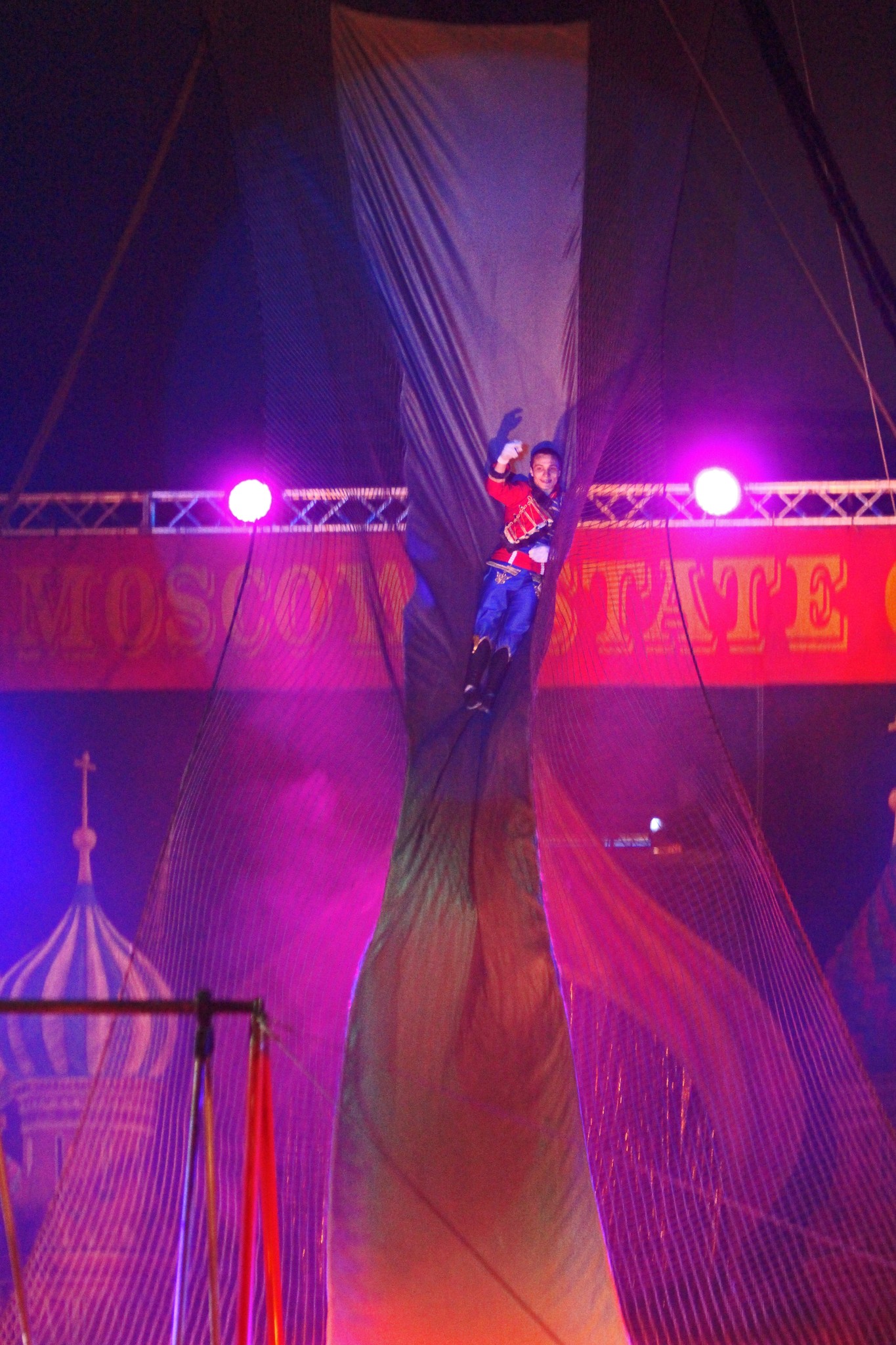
[528,539,549,565]
[496,438,524,465]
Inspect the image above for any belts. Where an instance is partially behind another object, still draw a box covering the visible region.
[485,560,542,583]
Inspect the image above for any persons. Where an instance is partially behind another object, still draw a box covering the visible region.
[460,441,566,713]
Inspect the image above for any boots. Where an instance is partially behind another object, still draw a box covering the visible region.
[463,634,491,709]
[478,645,511,714]
